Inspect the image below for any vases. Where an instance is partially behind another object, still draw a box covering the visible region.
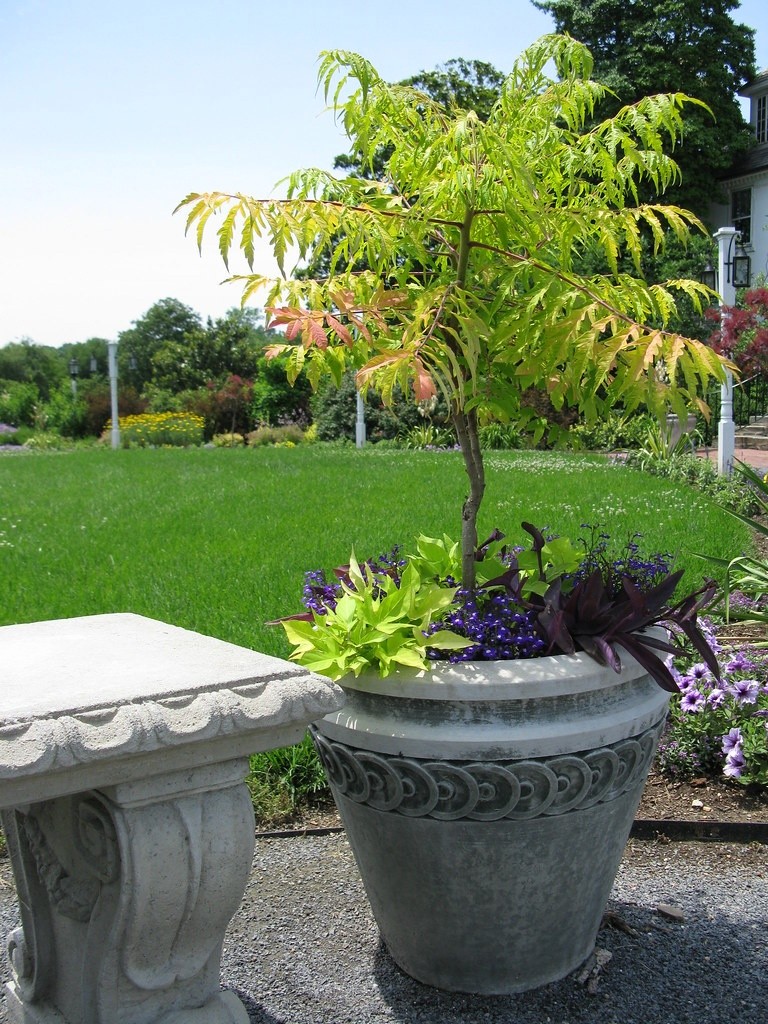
[290,620,672,996]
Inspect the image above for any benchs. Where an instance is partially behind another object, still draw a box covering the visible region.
[1,610,345,1024]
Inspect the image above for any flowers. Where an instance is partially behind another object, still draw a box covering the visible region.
[266,523,723,695]
